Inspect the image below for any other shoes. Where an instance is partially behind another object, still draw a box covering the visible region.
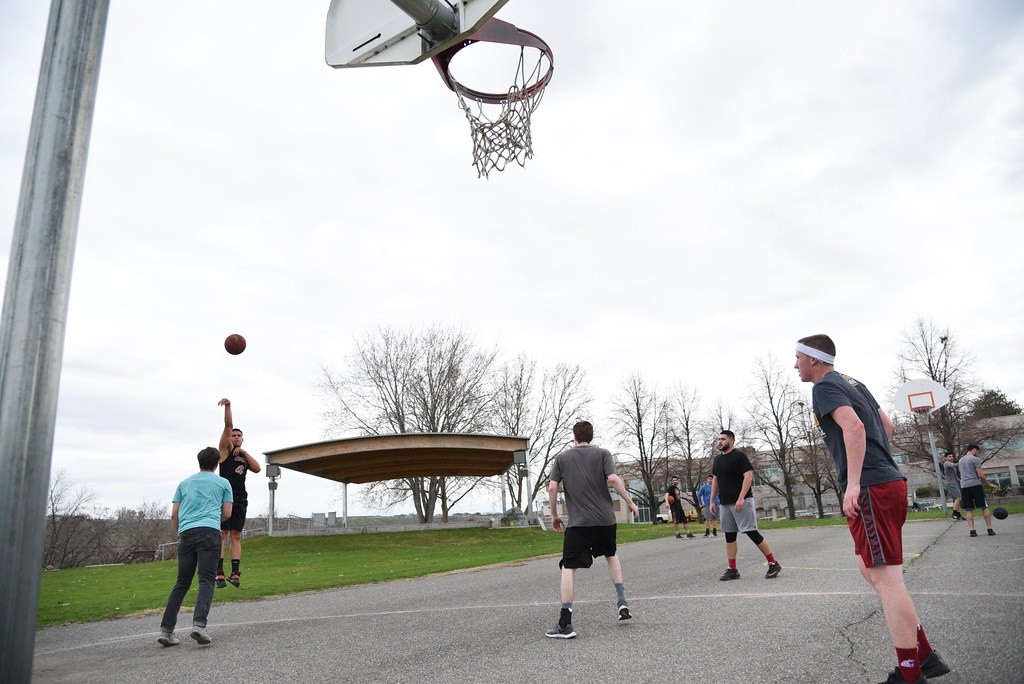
[687,533,696,537]
[951,511,966,520]
[703,534,711,538]
[713,534,717,538]
[675,535,686,540]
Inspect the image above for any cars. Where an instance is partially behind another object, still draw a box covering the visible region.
[795,510,811,518]
[813,511,832,518]
[685,510,706,521]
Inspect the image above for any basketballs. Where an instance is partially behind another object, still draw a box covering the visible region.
[225,334,246,355]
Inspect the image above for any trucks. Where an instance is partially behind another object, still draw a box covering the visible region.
[656,509,672,524]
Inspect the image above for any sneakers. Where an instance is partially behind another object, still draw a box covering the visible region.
[545,623,577,639]
[157,631,179,646]
[190,625,211,644]
[720,568,740,581]
[919,649,950,679]
[988,529,996,535]
[225,572,240,588]
[765,561,781,578]
[879,665,927,684]
[970,530,977,537]
[214,571,227,587]
[616,599,631,620]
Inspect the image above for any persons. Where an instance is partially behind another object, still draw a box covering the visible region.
[157,447,233,647]
[710,430,781,580]
[545,421,639,638]
[793,334,950,684]
[943,453,966,520]
[215,398,260,588]
[698,475,720,537]
[664,476,696,539]
[958,445,998,536]
